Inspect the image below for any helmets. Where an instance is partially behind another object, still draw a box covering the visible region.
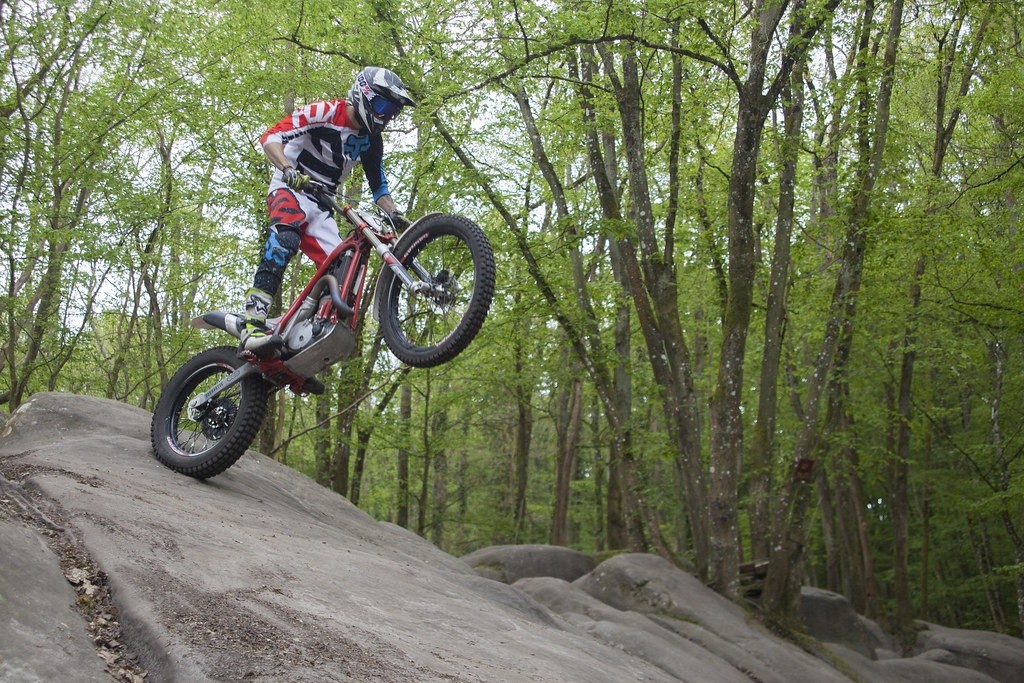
[350,66,418,137]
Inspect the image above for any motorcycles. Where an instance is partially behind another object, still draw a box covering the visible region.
[150,174,497,482]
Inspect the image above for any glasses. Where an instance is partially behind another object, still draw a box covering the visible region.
[369,94,402,119]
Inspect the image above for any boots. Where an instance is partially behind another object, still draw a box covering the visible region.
[237,288,284,359]
[304,376,325,395]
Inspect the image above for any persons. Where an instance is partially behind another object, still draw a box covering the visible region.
[237,66,418,359]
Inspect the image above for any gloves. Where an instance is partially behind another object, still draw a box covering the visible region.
[283,166,311,195]
[393,215,412,237]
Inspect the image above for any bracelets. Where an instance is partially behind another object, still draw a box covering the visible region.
[282,165,293,173]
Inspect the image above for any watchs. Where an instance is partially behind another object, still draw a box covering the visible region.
[388,209,403,216]
